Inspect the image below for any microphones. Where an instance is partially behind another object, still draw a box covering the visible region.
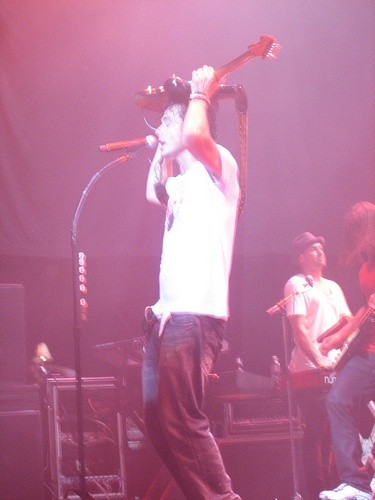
[99,135,158,152]
[305,275,314,287]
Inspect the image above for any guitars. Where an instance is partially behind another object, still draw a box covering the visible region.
[133,35,282,117]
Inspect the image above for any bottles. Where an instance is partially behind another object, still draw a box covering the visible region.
[234,352,242,371]
[270,355,281,392]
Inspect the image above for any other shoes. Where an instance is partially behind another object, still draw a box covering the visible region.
[309,474,340,490]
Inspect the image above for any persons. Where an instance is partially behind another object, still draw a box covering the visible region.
[284,231,359,500]
[319,200,375,500]
[142,64,240,499]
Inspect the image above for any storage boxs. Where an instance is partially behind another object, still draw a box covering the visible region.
[45,377,127,500]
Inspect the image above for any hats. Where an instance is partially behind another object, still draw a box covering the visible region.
[292,233,326,266]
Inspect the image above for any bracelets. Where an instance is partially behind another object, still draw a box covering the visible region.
[189,91,210,105]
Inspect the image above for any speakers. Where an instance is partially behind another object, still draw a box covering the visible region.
[0,283,47,500]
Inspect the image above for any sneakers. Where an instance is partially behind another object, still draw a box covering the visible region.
[319,483,373,500]
[370,478,375,491]
